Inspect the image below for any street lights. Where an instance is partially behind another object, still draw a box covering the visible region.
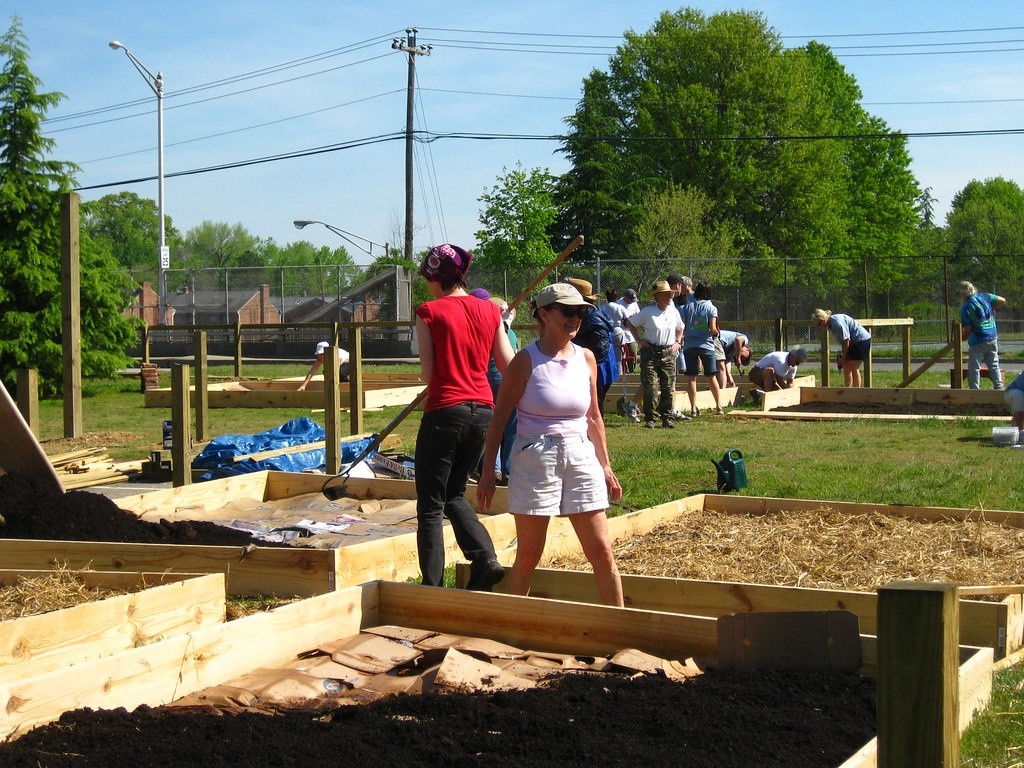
[294,221,389,261]
[109,40,164,321]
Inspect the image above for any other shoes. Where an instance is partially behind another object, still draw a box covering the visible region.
[691,411,696,417]
[502,475,509,486]
[496,474,501,485]
[716,406,724,414]
[465,560,506,592]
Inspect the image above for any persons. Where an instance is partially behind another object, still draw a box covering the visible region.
[748,344,808,392]
[414,245,521,590]
[476,281,624,610]
[296,341,349,392]
[811,308,871,388]
[953,278,1007,391]
[567,275,621,424]
[625,272,724,429]
[599,288,641,375]
[712,330,752,388]
[1003,369,1024,433]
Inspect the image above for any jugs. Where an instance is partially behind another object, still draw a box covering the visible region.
[710,449,748,492]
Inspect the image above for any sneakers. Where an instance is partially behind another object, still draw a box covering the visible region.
[623,402,640,423]
[662,419,675,428]
[645,421,656,429]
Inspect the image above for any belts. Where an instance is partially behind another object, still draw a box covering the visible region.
[461,403,491,409]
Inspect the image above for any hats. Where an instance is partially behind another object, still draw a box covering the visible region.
[469,289,490,300]
[534,283,597,311]
[811,308,832,322]
[649,281,678,299]
[741,346,753,366]
[567,278,597,300]
[682,276,692,286]
[625,289,639,302]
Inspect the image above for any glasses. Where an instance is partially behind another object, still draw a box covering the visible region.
[550,305,586,320]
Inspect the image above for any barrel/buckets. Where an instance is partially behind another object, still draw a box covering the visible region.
[162,420,173,449]
[993,427,1019,447]
